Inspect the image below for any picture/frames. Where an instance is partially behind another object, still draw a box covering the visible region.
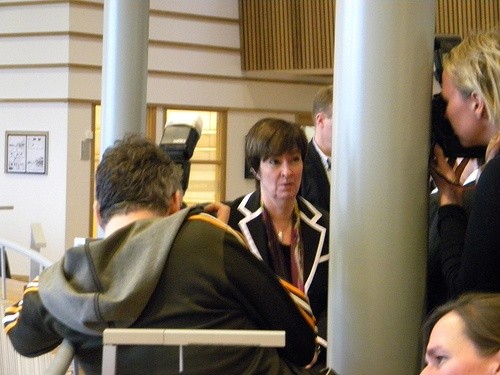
[4,130,49,175]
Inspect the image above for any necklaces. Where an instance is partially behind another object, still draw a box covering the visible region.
[273,218,291,242]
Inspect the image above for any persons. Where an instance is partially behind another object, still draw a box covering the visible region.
[298,84,334,212]
[426,27,500,327]
[220,117,330,369]
[3,131,319,375]
[415,294,500,375]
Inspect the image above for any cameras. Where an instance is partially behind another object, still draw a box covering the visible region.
[157,115,205,211]
[428,35,488,161]
